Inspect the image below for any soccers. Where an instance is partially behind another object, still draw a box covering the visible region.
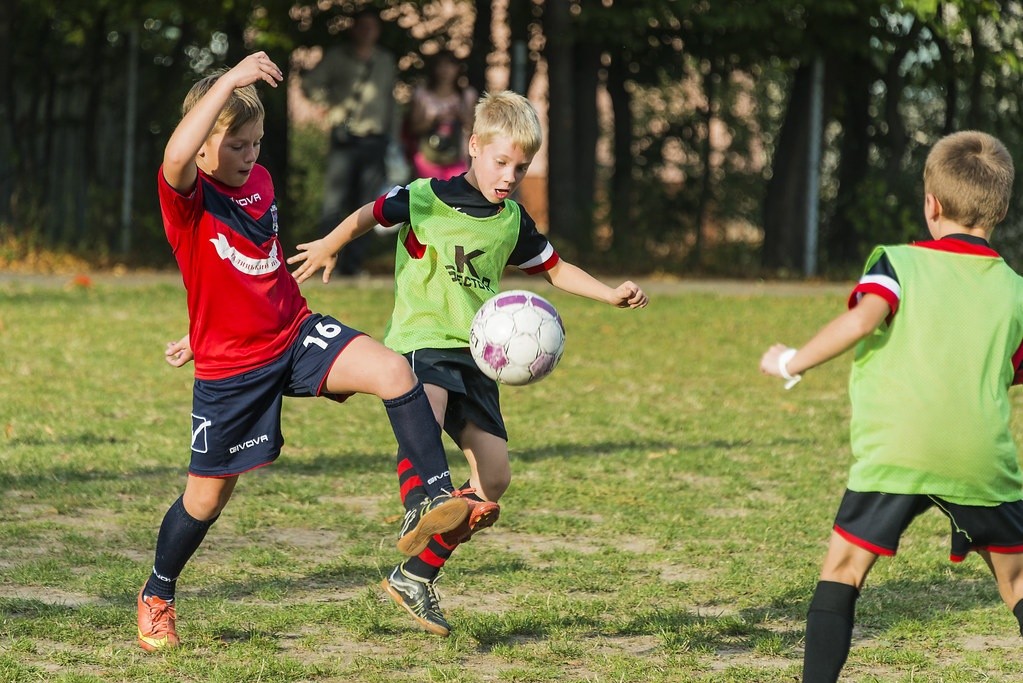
[467,290,566,384]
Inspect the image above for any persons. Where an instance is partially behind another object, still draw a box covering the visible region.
[404,51,478,181]
[761,129,1023,683]
[135,52,502,653]
[296,12,410,273]
[284,88,650,635]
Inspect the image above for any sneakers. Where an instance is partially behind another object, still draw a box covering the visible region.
[137,580,180,654]
[440,488,501,547]
[396,494,469,556]
[381,561,451,637]
